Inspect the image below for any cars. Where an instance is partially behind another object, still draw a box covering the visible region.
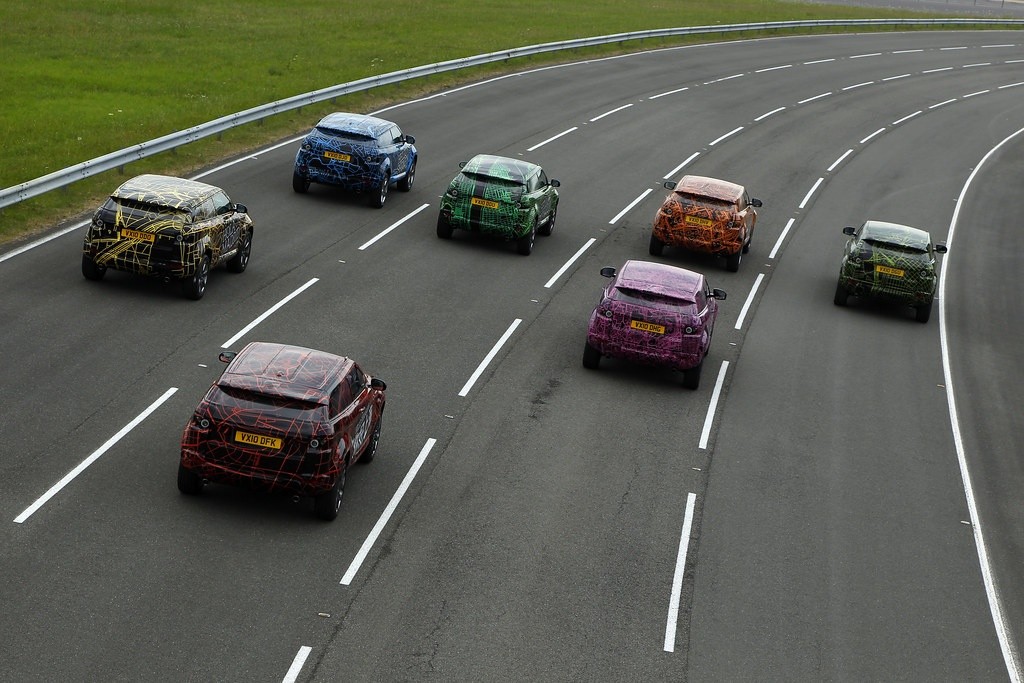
[834,218,948,323]
[648,175,763,274]
[177,341,385,521]
[584,259,729,391]
[82,173,252,302]
[437,153,562,257]
[293,110,419,209]
